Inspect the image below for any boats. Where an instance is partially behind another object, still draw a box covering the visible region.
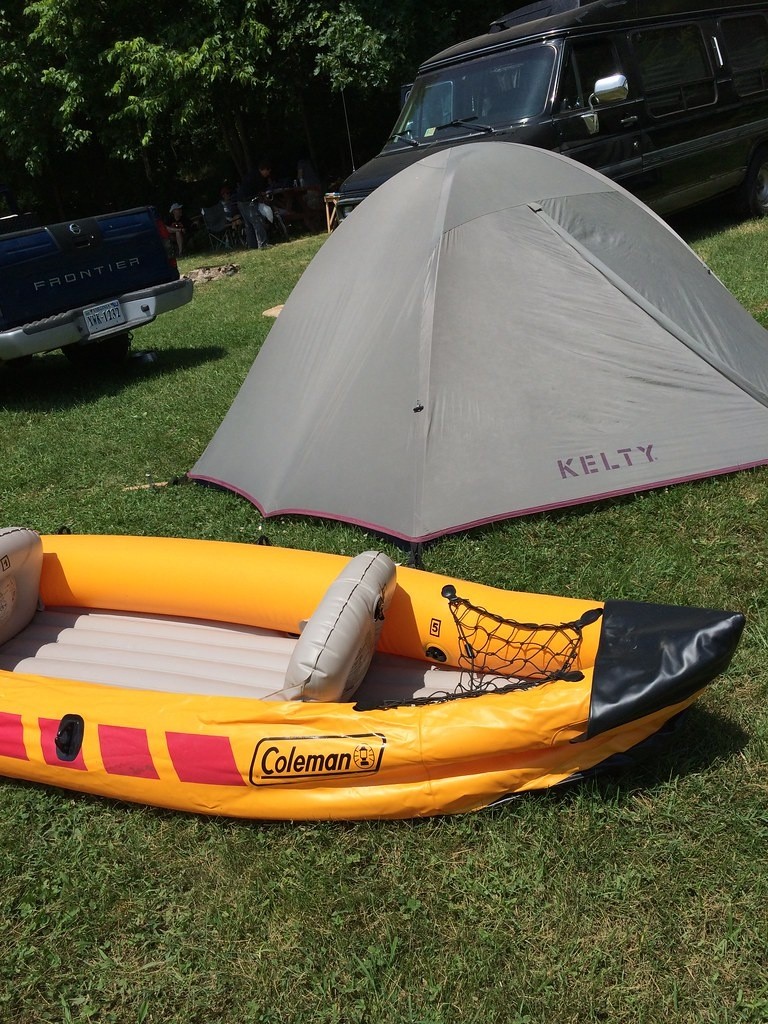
[0,526,747,823]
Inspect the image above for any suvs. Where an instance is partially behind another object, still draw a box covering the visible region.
[324,0,768,232]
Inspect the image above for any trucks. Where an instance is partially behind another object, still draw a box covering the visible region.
[0,204,199,363]
